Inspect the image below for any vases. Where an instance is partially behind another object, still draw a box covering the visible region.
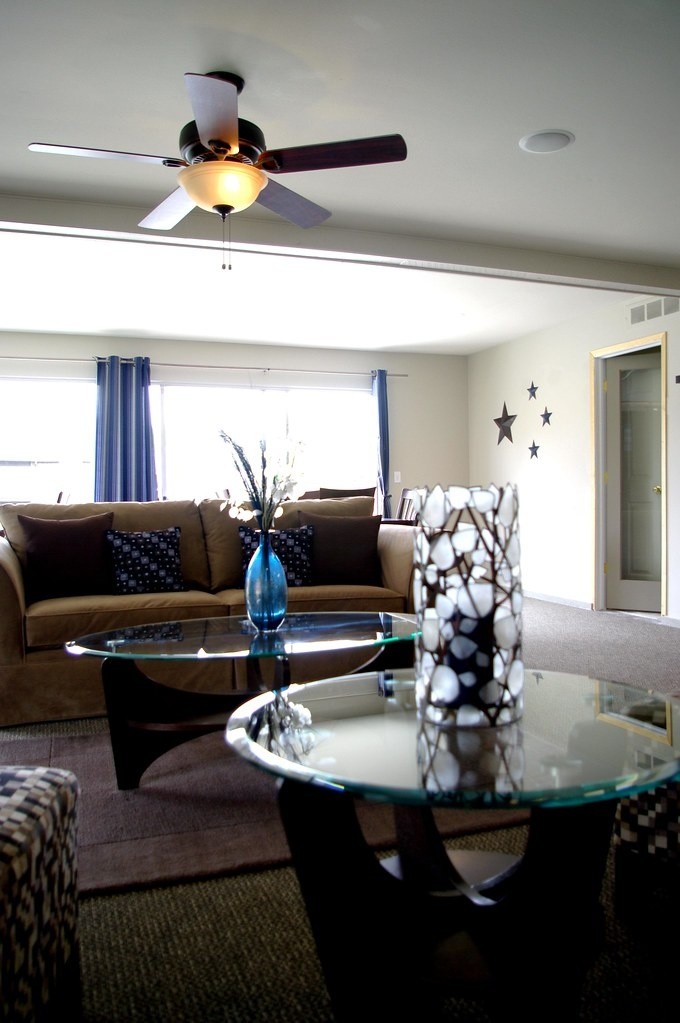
[411,484,527,728]
[245,529,288,632]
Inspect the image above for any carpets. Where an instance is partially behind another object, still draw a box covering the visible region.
[0,733,532,893]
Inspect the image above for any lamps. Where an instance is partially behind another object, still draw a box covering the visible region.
[177,160,268,222]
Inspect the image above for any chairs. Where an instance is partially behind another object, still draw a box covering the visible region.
[398,486,428,520]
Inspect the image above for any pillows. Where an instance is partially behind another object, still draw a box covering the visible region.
[240,525,313,586]
[17,512,121,603]
[107,526,186,595]
[298,510,383,587]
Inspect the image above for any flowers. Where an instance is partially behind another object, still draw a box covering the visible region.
[212,425,302,625]
[256,693,315,765]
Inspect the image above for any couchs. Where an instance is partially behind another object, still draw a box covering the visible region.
[0,494,418,725]
[608,692,679,881]
[0,765,89,1023]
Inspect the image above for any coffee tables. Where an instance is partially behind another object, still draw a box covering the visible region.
[227,665,680,1023]
[63,611,417,791]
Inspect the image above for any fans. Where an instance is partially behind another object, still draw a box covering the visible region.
[27,71,407,232]
